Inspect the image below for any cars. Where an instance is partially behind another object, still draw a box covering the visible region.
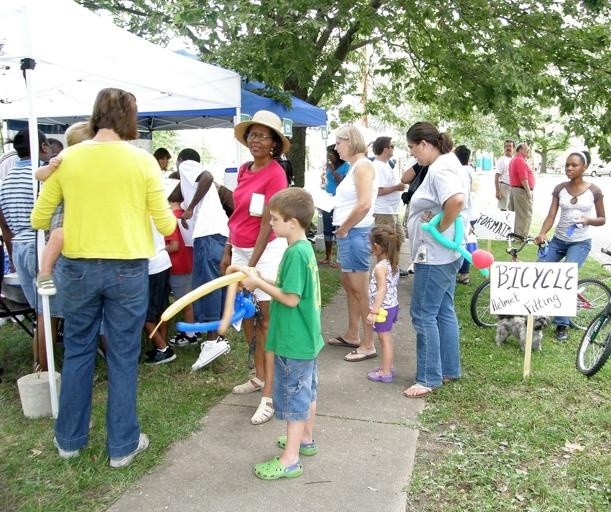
[582,164,610,177]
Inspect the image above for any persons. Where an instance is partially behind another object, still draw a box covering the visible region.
[510,144,535,243]
[321,122,477,399]
[534,151,605,340]
[495,140,515,211]
[0,88,325,482]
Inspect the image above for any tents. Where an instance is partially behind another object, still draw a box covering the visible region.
[1,1,328,416]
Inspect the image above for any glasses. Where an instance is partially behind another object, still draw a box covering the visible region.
[327,152,335,156]
[383,146,395,149]
[248,133,273,141]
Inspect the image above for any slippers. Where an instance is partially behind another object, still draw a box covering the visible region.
[344,349,379,361]
[403,381,433,399]
[368,366,397,384]
[328,334,362,348]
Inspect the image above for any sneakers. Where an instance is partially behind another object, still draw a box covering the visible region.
[407,263,415,274]
[51,435,78,459]
[0,312,23,323]
[169,331,202,345]
[191,339,231,372]
[399,268,407,278]
[107,433,150,469]
[554,323,570,343]
[142,346,177,367]
[524,234,535,244]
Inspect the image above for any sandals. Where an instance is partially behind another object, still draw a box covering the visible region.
[330,260,340,270]
[275,434,318,457]
[252,456,304,480]
[230,378,270,394]
[456,275,470,285]
[243,397,277,426]
[317,257,333,265]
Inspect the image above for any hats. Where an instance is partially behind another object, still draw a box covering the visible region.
[234,110,288,154]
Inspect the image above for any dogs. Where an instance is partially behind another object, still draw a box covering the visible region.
[494,315,548,352]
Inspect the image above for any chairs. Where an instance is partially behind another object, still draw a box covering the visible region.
[0,245,35,382]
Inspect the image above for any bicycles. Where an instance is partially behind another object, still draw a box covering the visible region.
[575,248,611,377]
[470,232,611,331]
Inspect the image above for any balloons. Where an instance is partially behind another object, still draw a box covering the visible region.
[472,248,493,269]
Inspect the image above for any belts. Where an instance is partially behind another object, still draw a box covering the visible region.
[511,184,534,191]
